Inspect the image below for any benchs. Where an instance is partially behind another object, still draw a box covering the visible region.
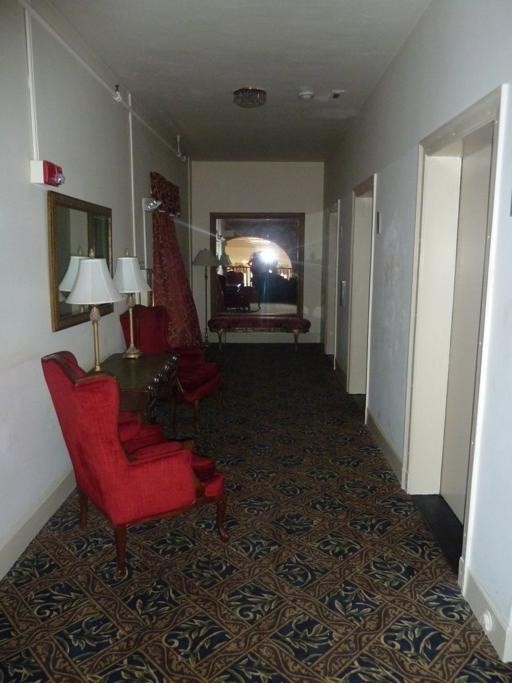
[208,317,311,352]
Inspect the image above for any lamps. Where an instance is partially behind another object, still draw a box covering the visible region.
[58,247,88,292]
[115,248,152,360]
[233,89,267,109]
[192,249,221,347]
[65,249,125,372]
[219,253,231,279]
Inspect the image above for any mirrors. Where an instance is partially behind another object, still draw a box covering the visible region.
[210,212,305,317]
[47,190,115,332]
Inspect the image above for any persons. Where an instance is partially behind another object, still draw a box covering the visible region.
[246,246,280,300]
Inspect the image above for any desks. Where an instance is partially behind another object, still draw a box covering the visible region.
[90,352,180,434]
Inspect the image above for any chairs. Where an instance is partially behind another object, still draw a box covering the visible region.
[120,304,225,434]
[229,271,262,311]
[41,352,231,579]
